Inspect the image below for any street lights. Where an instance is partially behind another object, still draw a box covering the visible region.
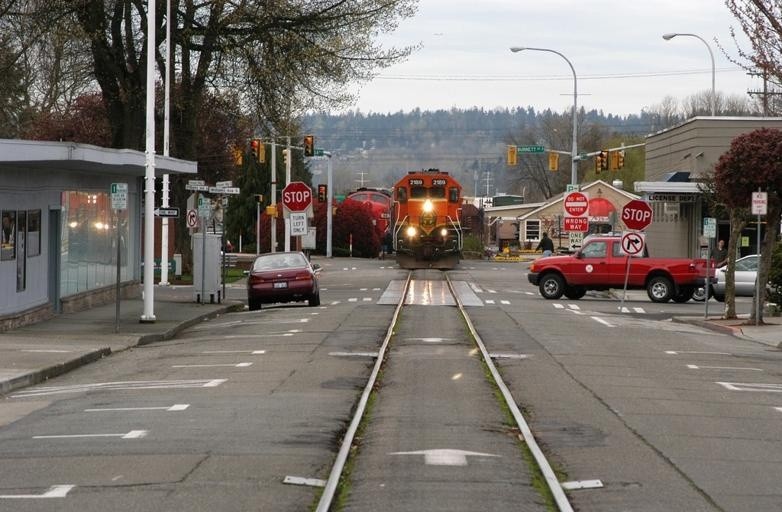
[511,44,581,252]
[664,31,718,117]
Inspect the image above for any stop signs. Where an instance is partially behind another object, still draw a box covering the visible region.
[563,192,589,232]
[621,199,654,233]
[282,181,312,212]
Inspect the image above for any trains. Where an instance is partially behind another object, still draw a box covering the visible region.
[387,168,465,272]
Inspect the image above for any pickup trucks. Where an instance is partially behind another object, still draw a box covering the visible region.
[527,235,717,304]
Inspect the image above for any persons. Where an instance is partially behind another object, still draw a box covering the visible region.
[1,212,14,246]
[535,232,554,257]
[710,240,728,269]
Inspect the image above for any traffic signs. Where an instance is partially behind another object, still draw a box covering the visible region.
[569,232,584,252]
[314,148,324,156]
[517,145,544,154]
[153,207,180,219]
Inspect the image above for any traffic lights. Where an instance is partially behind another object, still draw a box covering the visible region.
[601,149,609,170]
[596,156,602,174]
[612,151,625,170]
[282,149,291,165]
[254,195,263,202]
[304,136,314,156]
[318,184,327,202]
[252,138,260,160]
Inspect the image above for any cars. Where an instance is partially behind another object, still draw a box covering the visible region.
[554,230,624,258]
[242,251,321,311]
[219,238,231,253]
[692,253,782,301]
